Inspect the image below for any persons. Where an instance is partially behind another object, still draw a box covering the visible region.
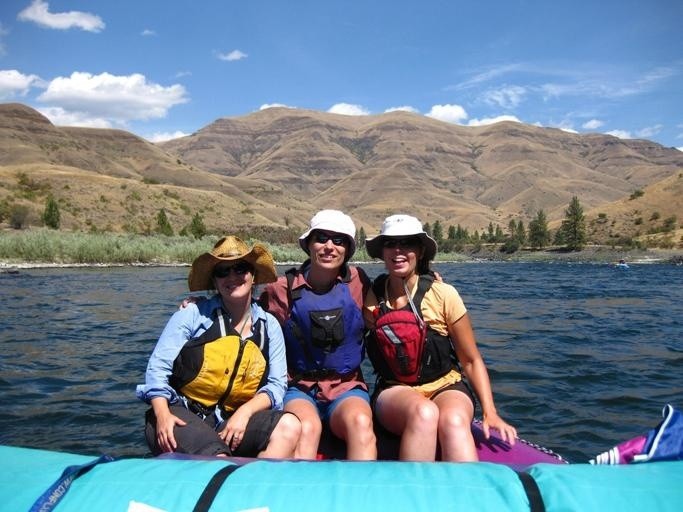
[179,208,444,460]
[136,234,302,459]
[362,214,517,465]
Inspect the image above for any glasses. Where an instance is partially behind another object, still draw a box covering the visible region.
[316,231,346,246]
[383,239,417,249]
[212,264,248,277]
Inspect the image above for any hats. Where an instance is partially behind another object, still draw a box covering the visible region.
[365,215,438,263]
[298,210,356,262]
[189,237,278,291]
